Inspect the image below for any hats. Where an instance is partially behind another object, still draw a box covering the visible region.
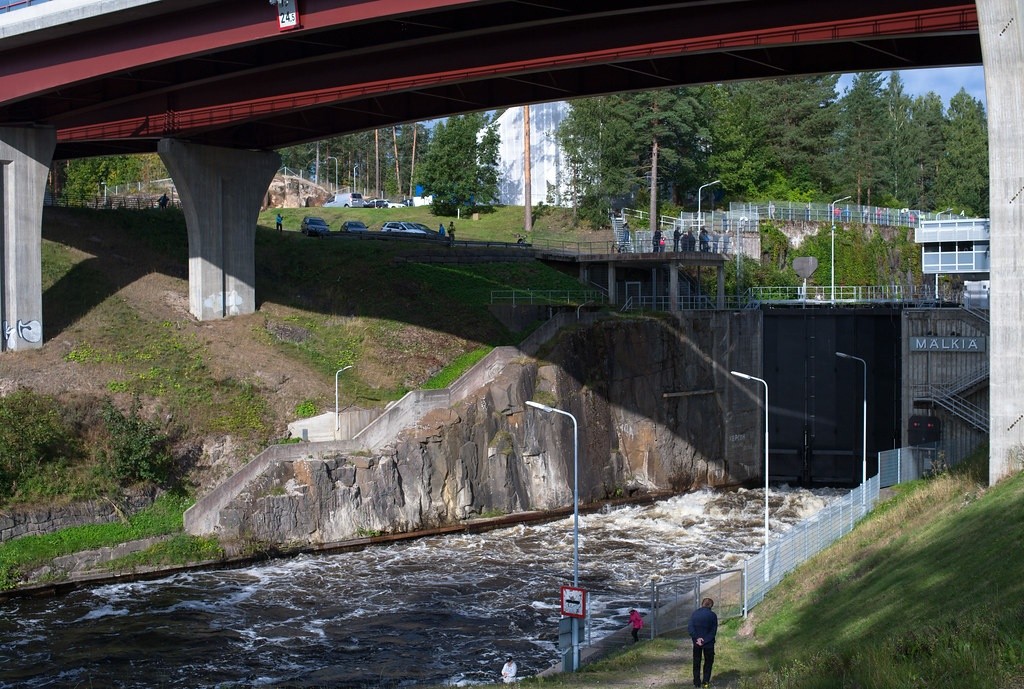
[505,656,512,661]
[625,607,633,612]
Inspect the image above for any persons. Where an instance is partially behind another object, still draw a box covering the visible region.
[653,226,709,252]
[622,223,630,242]
[276,214,283,232]
[628,607,644,642]
[688,598,718,689]
[501,656,517,684]
[773,203,926,229]
[439,224,445,241]
[156,193,170,209]
[447,221,457,248]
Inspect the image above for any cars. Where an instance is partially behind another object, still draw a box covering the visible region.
[341,220,370,231]
[363,197,388,208]
[384,199,406,208]
[380,222,427,235]
[400,199,413,206]
[301,216,330,235]
[414,223,440,236]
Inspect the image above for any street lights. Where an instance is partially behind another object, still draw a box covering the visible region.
[354,166,358,193]
[696,180,721,251]
[334,366,352,440]
[935,208,954,300]
[836,352,867,515]
[731,371,770,584]
[328,157,338,194]
[525,400,580,673]
[830,195,852,300]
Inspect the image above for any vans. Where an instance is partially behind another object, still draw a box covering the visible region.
[322,192,364,208]
